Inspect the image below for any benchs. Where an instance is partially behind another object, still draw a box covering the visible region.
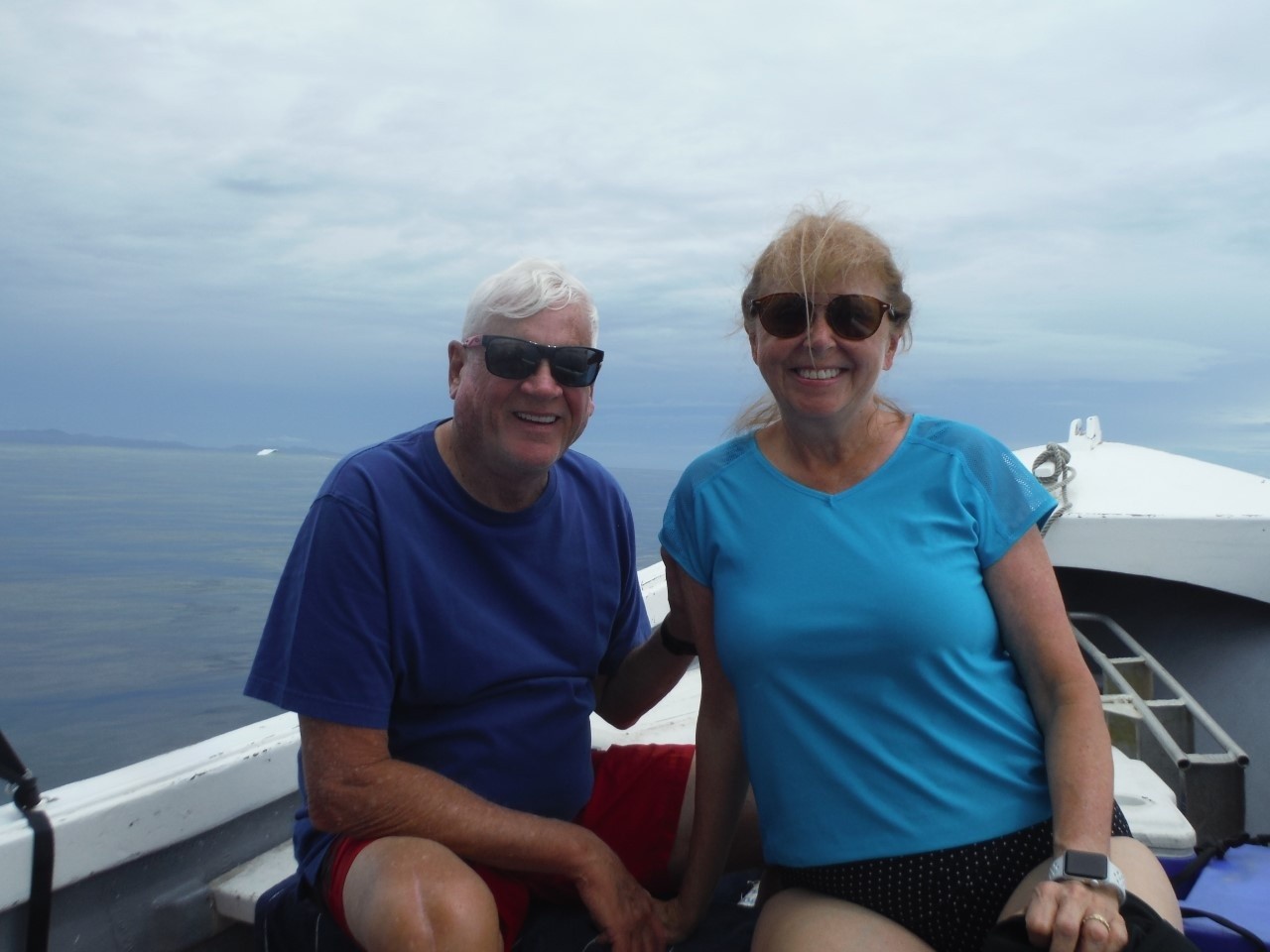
[211,649,1194,926]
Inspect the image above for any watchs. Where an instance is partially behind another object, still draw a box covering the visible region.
[1045,849,1125,907]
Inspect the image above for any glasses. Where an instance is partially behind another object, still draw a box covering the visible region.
[462,333,609,388]
[747,291,904,341]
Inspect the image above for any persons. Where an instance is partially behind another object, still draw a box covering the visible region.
[656,214,1185,952]
[239,263,699,952]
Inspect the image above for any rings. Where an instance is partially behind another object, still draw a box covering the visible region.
[1081,914,1111,933]
